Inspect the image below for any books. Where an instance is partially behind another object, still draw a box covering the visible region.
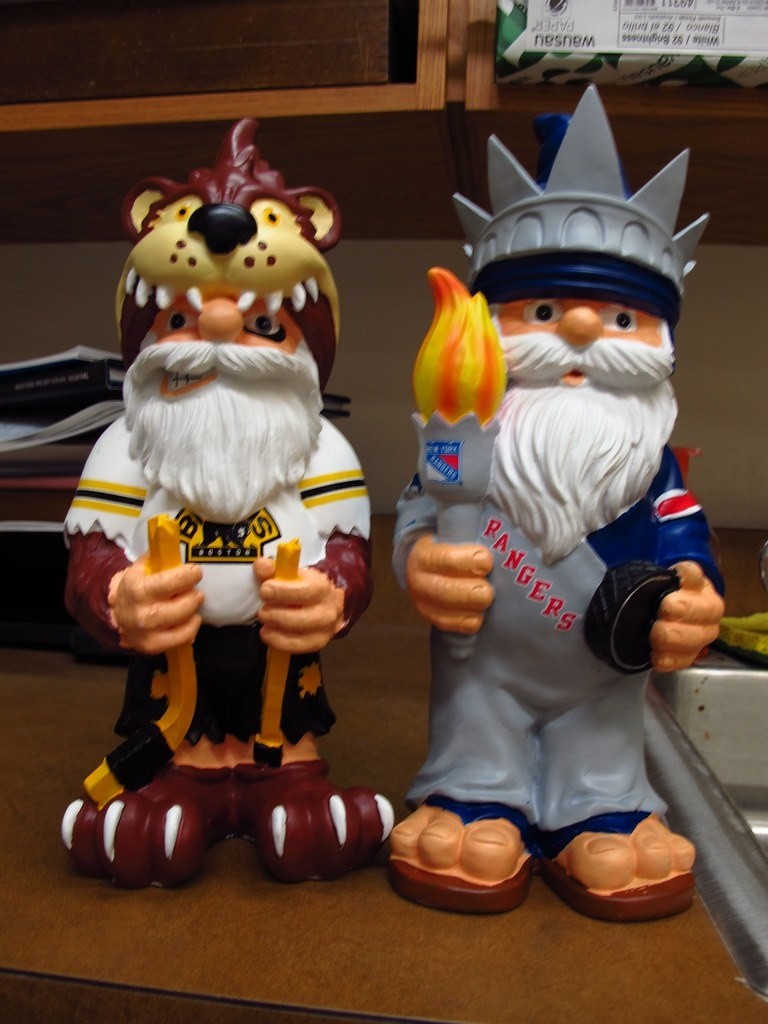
[0,343,349,451]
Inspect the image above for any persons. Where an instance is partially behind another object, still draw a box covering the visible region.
[394,79,728,920]
[57,114,394,887]
